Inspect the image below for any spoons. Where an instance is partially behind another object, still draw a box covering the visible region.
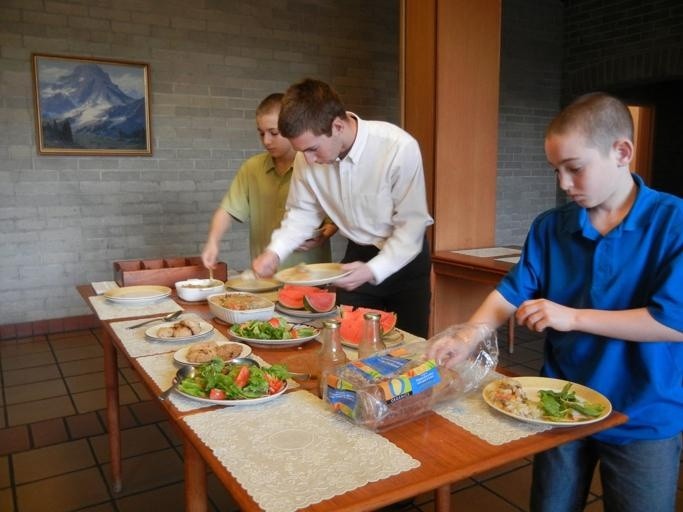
[128,310,182,330]
[207,266,214,286]
[159,365,193,402]
[227,357,313,381]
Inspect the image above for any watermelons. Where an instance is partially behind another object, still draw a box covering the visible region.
[339,304,398,346]
[274,283,337,314]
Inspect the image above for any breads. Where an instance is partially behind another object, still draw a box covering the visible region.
[155,318,201,337]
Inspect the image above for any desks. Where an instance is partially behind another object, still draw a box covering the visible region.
[75,278,630,510]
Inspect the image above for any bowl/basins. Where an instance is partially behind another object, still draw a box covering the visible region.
[208,291,272,324]
[174,278,221,301]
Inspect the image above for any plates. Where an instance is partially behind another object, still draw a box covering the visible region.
[225,277,283,293]
[275,305,337,318]
[274,262,352,287]
[300,228,325,241]
[174,341,251,364]
[102,283,171,304]
[482,375,613,425]
[227,323,321,349]
[173,376,288,401]
[341,329,404,351]
[148,320,211,342]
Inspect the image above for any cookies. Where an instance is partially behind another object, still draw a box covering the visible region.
[186,339,243,363]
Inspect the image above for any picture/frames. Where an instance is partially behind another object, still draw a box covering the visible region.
[30,52,154,158]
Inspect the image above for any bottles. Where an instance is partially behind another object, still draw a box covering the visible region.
[318,318,348,397]
[356,314,384,361]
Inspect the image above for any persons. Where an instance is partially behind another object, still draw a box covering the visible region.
[201,93,340,276]
[249,79,435,509]
[424,92,682,512]
